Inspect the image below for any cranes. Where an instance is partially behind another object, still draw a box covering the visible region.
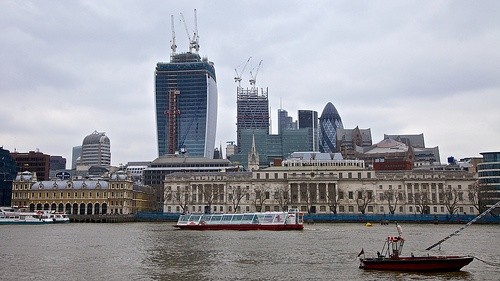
[233,56,263,92]
[170,8,200,56]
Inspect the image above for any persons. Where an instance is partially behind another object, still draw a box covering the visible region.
[252,215,259,223]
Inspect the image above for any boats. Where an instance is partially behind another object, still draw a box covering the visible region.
[357,220,474,272]
[173,211,304,230]
[0,211,69,224]
